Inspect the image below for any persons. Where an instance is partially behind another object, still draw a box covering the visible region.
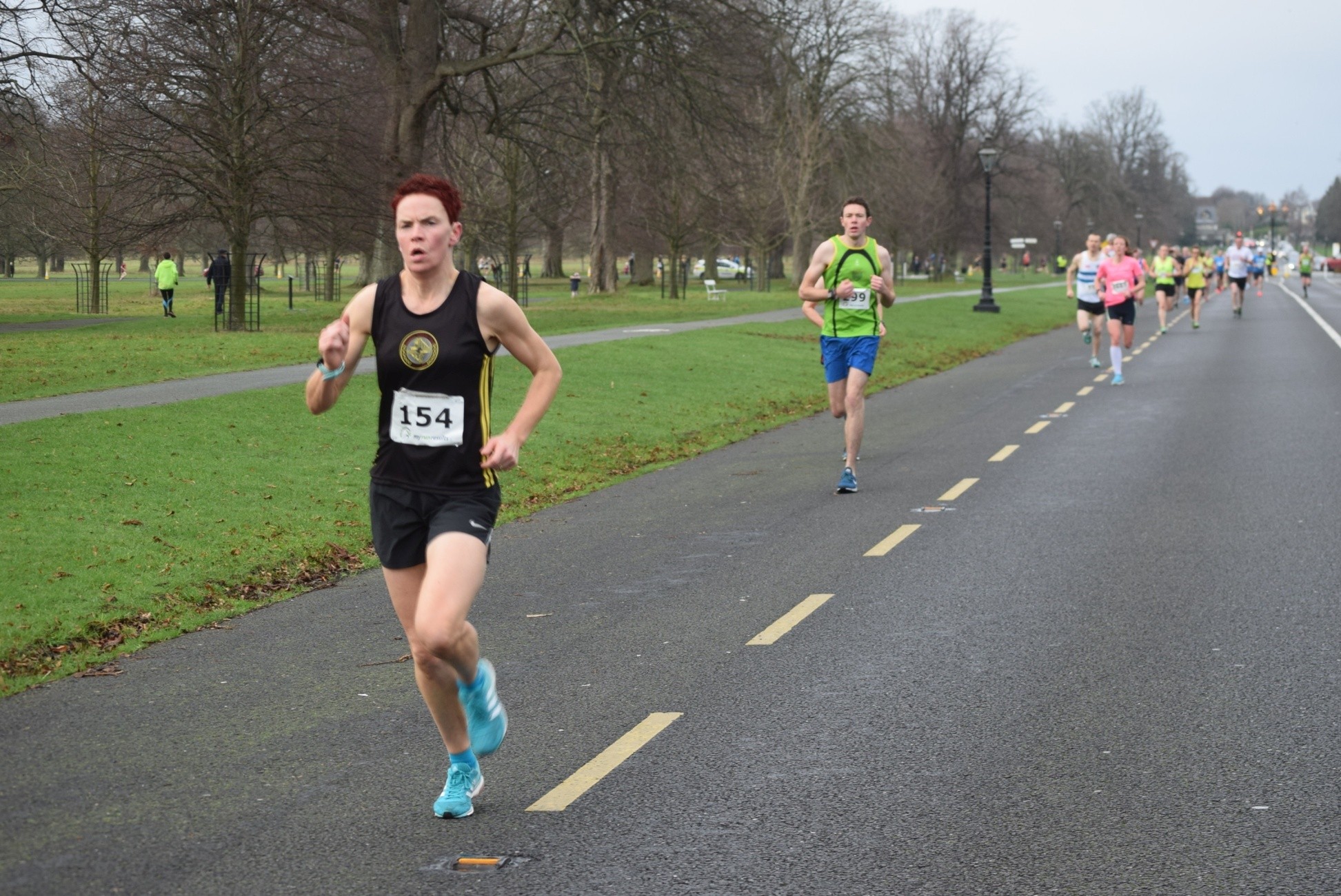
[154,251,179,318]
[10,262,14,278]
[797,196,1313,493]
[207,249,230,314]
[119,261,127,281]
[571,252,751,297]
[304,175,563,819]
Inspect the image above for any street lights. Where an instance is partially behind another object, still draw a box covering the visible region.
[1087,217,1094,234]
[974,133,1001,312]
[1256,201,1288,253]
[1054,216,1063,273]
[1134,208,1143,247]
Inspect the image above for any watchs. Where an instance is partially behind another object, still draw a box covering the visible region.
[316,357,345,381]
[827,288,835,299]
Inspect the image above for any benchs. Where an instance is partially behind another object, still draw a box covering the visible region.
[955,271,964,282]
[704,280,728,302]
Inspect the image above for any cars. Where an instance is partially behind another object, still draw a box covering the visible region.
[1320,253,1341,273]
[1244,239,1256,248]
[693,259,754,280]
[1312,256,1326,270]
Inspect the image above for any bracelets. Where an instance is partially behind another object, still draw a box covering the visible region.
[834,288,837,299]
[1129,290,1133,295]
[879,320,884,325]
[1096,289,1101,294]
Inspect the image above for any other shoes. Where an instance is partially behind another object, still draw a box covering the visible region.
[1161,326,1167,333]
[1192,321,1200,329]
[1233,306,1242,315]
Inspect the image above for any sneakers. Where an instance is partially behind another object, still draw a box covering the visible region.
[1090,358,1101,368]
[1084,331,1091,344]
[458,660,507,756]
[837,467,858,492]
[434,761,485,819]
[843,448,860,461]
[1111,375,1125,385]
